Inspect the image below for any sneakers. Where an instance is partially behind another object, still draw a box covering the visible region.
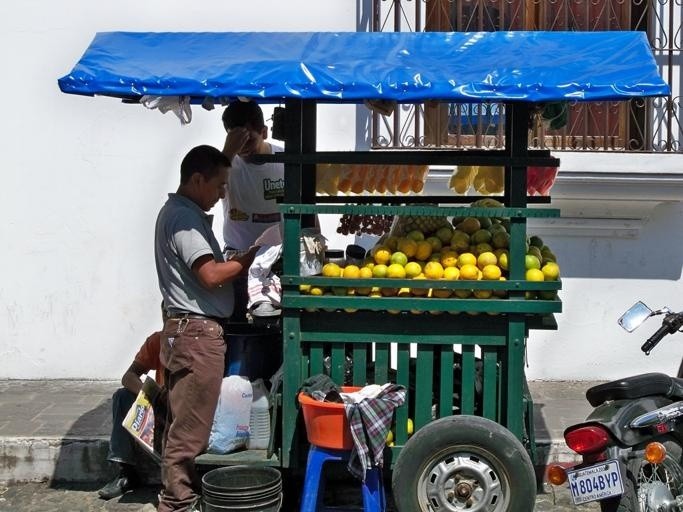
[99,467,142,498]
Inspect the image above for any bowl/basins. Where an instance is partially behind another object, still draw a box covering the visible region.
[298,387,363,450]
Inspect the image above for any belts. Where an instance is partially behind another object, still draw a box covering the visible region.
[176,314,225,326]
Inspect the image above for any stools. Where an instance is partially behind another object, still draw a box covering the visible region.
[299,444,386,511]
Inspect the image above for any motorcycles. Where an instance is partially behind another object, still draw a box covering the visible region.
[545,300,682,511]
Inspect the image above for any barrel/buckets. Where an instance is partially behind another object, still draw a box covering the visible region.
[202,465,284,512]
[245,379,272,450]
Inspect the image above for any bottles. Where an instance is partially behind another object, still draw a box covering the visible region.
[324,244,366,265]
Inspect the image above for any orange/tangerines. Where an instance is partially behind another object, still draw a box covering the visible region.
[298,216,560,317]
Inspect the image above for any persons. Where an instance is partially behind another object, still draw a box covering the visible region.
[219,100,285,252]
[98,299,169,499]
[154,144,260,512]
